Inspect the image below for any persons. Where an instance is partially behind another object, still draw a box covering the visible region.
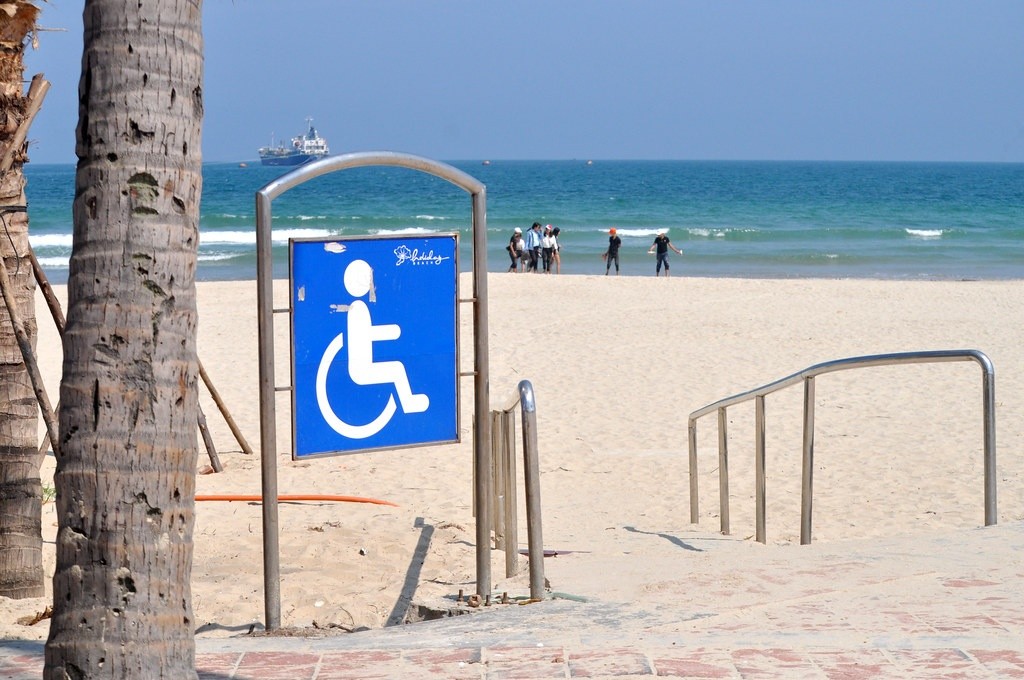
[504,222,542,274]
[550,227,562,274]
[602,227,622,275]
[540,223,557,274]
[648,229,683,277]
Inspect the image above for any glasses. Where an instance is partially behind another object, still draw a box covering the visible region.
[545,228,548,230]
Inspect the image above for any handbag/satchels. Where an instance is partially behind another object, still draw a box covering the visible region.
[506,246,511,251]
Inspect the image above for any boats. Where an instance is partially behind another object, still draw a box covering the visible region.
[258,115,329,166]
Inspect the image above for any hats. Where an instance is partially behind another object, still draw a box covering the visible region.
[546,224,552,229]
[656,229,663,235]
[608,228,616,234]
[515,227,522,233]
